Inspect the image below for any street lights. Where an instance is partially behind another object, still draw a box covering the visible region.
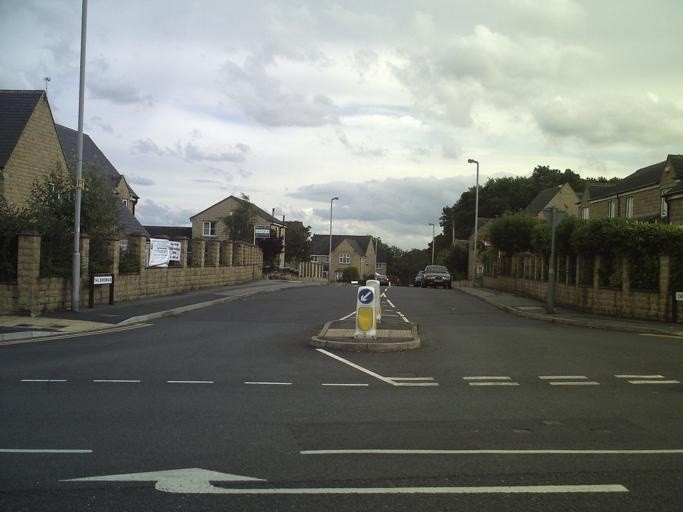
[327,196,339,282]
[427,222,435,265]
[466,158,480,289]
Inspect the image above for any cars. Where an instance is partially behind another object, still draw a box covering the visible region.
[388,275,401,286]
[413,270,424,286]
[376,274,389,286]
[421,264,452,290]
